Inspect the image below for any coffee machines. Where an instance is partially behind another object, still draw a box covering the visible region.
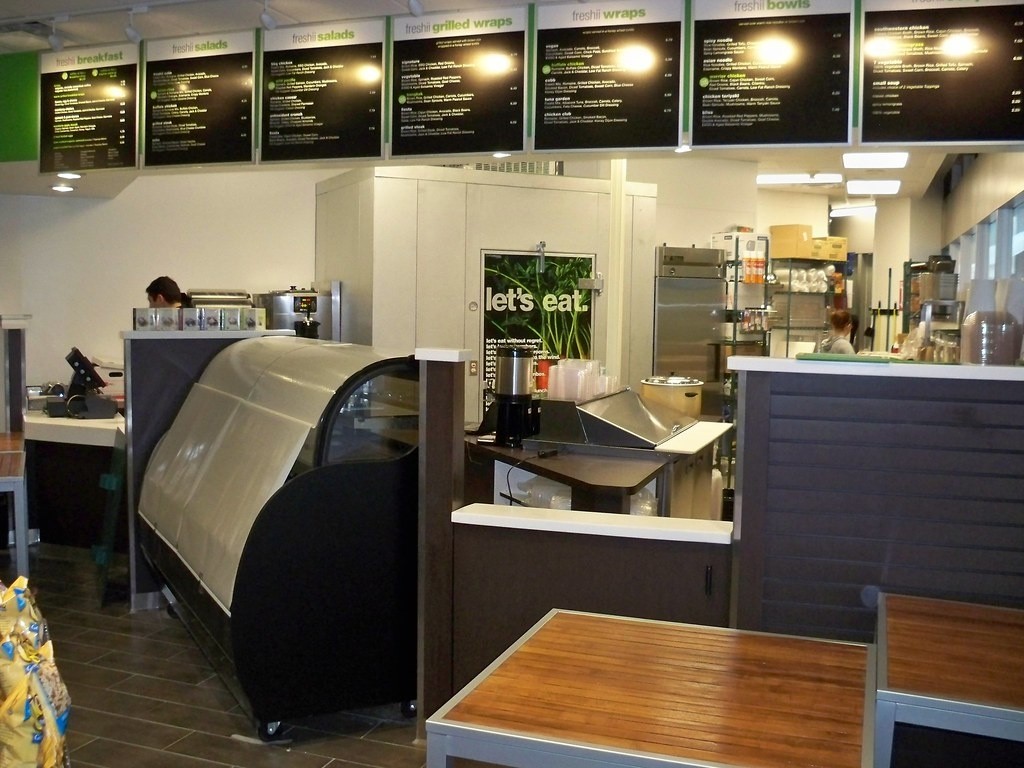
[478,343,538,448]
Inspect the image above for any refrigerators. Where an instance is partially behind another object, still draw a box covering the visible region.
[654,246,726,423]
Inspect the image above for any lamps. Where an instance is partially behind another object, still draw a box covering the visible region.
[124,5,149,45]
[407,1,425,19]
[259,1,277,31]
[47,15,70,54]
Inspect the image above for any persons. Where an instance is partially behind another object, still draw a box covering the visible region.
[146,275,183,308]
[820,307,856,355]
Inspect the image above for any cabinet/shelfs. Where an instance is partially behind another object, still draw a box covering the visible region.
[658,438,715,520]
[767,258,847,358]
[726,235,767,355]
[137,334,417,743]
[315,165,659,433]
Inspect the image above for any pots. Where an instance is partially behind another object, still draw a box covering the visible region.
[640,371,705,423]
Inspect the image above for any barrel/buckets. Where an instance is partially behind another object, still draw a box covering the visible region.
[722,486,735,522]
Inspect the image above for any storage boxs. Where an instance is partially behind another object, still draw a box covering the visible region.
[711,233,765,281]
[812,240,827,259]
[769,225,812,258]
[812,237,847,261]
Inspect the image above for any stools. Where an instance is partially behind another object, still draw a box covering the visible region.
[0,432,30,579]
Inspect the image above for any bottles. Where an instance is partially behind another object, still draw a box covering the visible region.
[743,310,770,331]
[742,253,766,283]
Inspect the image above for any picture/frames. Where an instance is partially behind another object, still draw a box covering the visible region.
[478,250,596,422]
